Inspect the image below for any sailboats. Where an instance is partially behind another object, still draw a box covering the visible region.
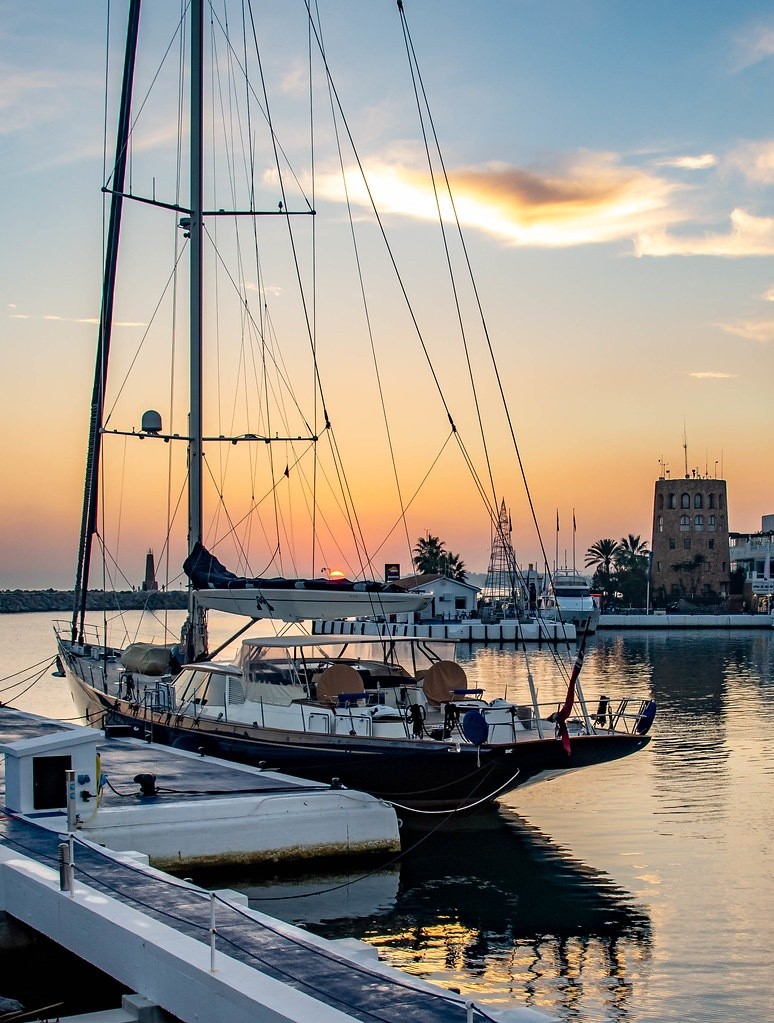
[50,1,660,803]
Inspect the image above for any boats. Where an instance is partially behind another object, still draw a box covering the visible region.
[538,507,602,635]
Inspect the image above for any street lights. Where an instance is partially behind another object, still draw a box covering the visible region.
[645,570,650,615]
